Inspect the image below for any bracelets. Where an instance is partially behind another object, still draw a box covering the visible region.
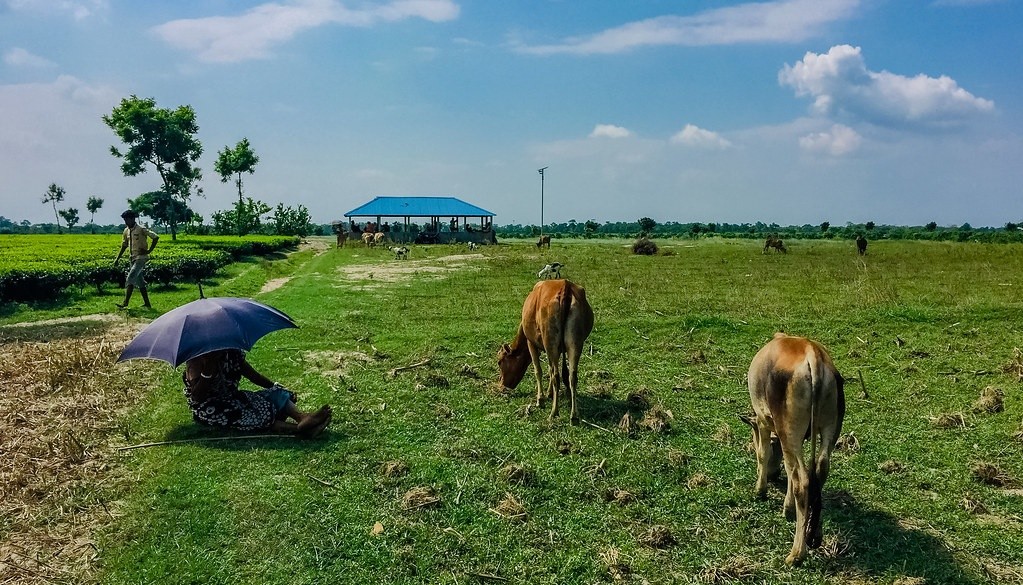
[200,372,212,379]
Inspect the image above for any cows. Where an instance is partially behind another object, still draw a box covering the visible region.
[761,238,789,255]
[737,333,846,570]
[336,231,479,260]
[496,279,594,426]
[856,236,868,256]
[536,235,551,249]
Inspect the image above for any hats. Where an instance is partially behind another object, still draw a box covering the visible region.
[121,210,139,218]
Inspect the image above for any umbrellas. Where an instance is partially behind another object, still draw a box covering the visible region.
[114,282,301,371]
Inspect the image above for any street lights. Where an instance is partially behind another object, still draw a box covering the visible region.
[537,166,549,235]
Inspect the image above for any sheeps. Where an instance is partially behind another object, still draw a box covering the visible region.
[536,261,564,280]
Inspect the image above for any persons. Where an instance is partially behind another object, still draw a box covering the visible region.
[113,210,159,307]
[336,217,491,249]
[184,349,334,438]
